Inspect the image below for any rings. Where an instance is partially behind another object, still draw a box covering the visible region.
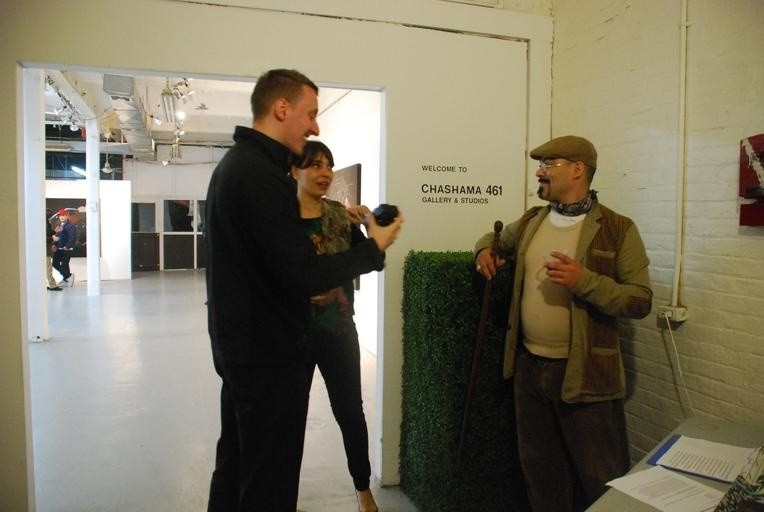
[476,265,481,273]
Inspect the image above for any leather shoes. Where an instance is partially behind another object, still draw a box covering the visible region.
[355,486,378,512]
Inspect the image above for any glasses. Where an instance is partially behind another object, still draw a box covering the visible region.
[537,159,573,173]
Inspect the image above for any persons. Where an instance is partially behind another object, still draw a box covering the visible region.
[471,134,654,512]
[287,141,380,511]
[201,67,404,511]
[45,210,64,291]
[49,209,76,288]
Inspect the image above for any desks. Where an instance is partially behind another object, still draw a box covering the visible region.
[584,416,763,512]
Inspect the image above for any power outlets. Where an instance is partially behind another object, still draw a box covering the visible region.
[658,306,688,322]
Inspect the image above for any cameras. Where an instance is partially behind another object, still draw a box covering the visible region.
[363,204,398,228]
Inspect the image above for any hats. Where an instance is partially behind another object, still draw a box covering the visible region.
[528,135,598,171]
[57,209,69,216]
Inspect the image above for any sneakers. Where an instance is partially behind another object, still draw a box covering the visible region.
[46,273,74,290]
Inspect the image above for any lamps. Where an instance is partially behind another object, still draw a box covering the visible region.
[45,76,82,133]
[149,78,208,161]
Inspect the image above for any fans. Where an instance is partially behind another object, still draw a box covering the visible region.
[100,129,127,174]
